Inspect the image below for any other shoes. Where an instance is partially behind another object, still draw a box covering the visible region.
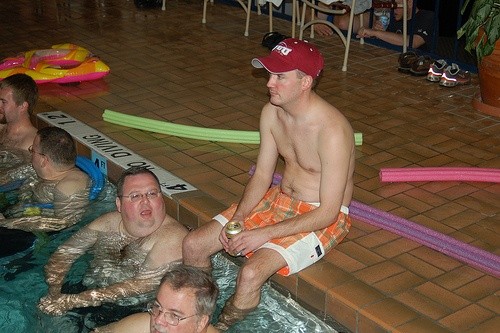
[397,51,417,74]
[409,55,434,77]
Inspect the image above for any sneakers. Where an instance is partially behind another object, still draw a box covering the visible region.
[439,62,472,88]
[426,58,449,82]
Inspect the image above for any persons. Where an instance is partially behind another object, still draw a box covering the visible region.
[0,74,41,149]
[182,37,356,308]
[298,0,376,37]
[118,264,222,333]
[28,126,90,195]
[356,0,436,53]
[84,166,190,266]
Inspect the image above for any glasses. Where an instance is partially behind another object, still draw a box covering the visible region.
[28,145,50,162]
[119,185,164,201]
[146,301,200,326]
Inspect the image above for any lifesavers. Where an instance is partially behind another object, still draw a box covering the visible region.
[248,163,500,280]
[21,153,107,215]
[100,108,366,147]
[378,164,500,188]
[0,42,112,85]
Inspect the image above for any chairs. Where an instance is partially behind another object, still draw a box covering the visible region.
[162,0,409,70]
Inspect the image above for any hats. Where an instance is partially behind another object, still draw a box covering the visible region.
[251,38,324,79]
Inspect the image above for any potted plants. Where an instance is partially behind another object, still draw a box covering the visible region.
[459,0,500,106]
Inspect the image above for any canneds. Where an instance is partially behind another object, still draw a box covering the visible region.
[379,15,389,31]
[225,221,243,256]
